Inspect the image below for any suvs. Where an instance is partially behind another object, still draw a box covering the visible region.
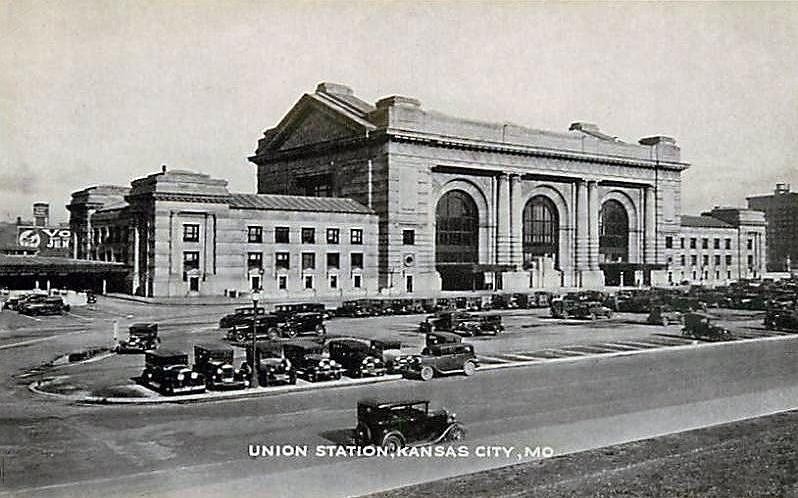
[354,397,467,452]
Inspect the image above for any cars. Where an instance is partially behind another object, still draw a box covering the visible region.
[4,292,63,316]
[116,278,798,395]
